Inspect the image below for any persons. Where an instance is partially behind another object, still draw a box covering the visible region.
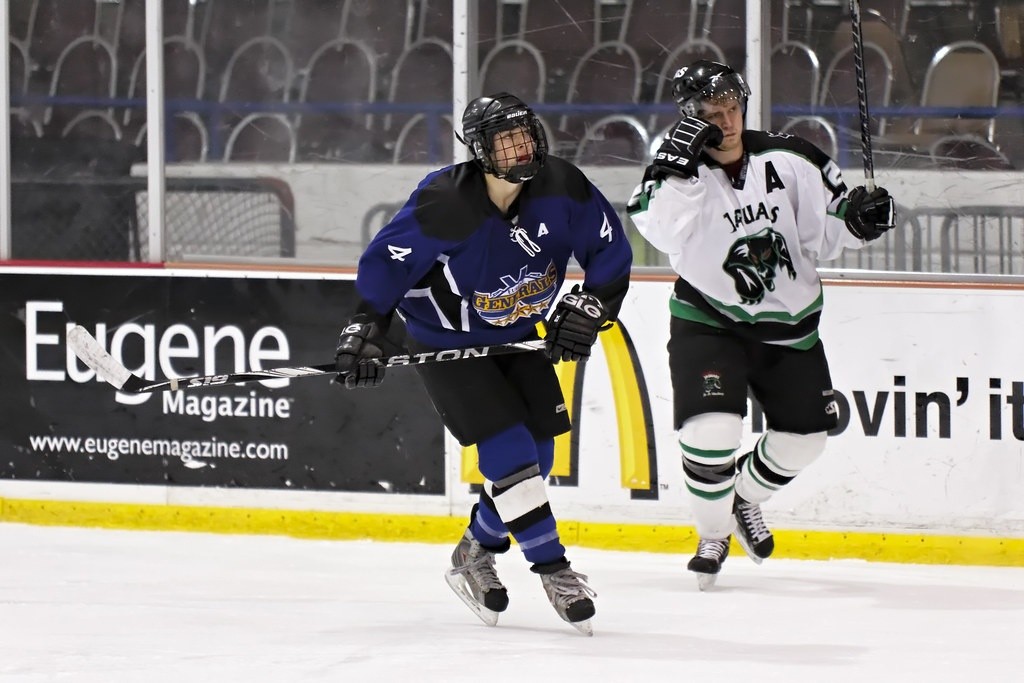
[336,91,633,638]
[625,59,895,591]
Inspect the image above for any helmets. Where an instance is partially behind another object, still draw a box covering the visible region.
[454,92,549,184]
[671,60,748,118]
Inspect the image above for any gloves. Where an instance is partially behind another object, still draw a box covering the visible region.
[544,283,613,365]
[653,115,724,179]
[845,185,897,241]
[333,313,385,389]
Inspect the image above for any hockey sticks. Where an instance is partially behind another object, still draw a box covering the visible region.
[846,0,877,196]
[66,323,549,398]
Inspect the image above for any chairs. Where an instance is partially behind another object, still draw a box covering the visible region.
[481,38,548,112]
[10,0,415,163]
[647,39,726,135]
[558,40,643,132]
[572,115,650,167]
[416,0,503,49]
[614,0,696,53]
[383,36,453,147]
[516,0,601,50]
[819,41,894,136]
[393,112,452,166]
[913,38,1001,154]
[769,39,821,128]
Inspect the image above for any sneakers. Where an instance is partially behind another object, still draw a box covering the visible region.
[688,533,732,592]
[731,491,774,565]
[529,555,597,637]
[444,502,508,627]
